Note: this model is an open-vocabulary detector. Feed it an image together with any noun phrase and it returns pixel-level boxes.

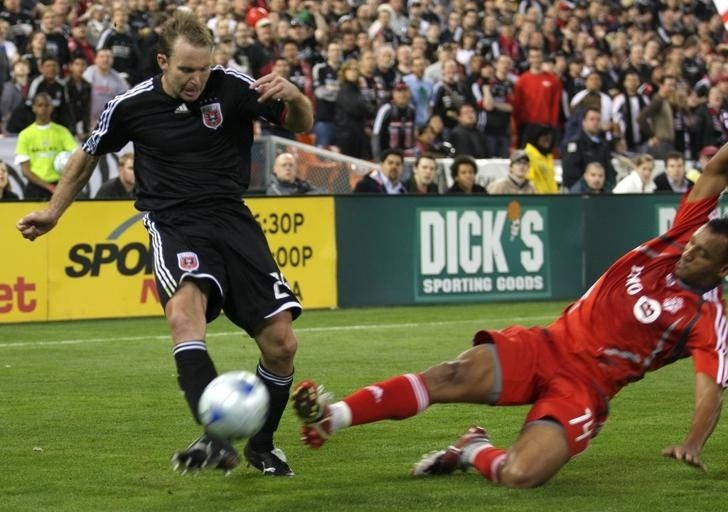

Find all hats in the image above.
[510,149,529,163]
[392,82,409,90]
[702,146,719,155]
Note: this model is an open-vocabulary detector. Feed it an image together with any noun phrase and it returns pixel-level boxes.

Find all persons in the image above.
[291,142,728,489]
[14,13,315,476]
[1,0,728,200]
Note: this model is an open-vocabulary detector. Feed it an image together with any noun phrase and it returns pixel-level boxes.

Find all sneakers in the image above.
[290,18,305,27]
[256,18,271,26]
[243,439,295,476]
[172,432,240,478]
[292,378,331,448]
[412,423,489,475]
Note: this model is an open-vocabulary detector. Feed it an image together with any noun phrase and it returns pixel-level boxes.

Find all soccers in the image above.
[198,371,270,441]
